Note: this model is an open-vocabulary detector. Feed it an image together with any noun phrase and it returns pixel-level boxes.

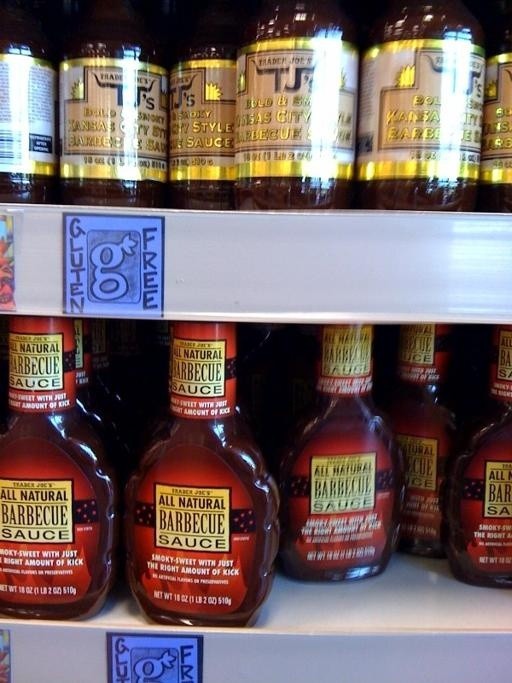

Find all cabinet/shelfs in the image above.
[0,200,511,683]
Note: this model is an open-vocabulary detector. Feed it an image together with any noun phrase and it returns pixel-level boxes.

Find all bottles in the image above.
[1,1,510,213]
[4,309,512,625]
